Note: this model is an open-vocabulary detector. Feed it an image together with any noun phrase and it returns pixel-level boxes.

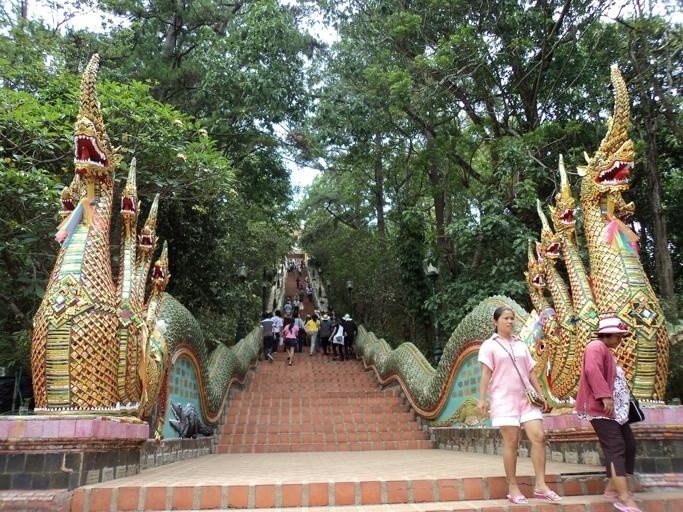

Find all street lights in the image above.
[422,246,443,366]
[345,279,355,315]
[324,279,335,312]
[234,261,247,345]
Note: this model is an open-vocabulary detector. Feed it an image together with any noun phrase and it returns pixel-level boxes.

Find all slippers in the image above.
[533,490,562,501]
[603,490,645,512]
[506,494,528,504]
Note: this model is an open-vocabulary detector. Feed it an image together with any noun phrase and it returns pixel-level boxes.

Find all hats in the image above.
[342,314,353,321]
[593,317,633,338]
[320,314,330,320]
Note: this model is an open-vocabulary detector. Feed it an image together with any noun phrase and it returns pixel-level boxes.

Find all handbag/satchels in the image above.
[627,399,645,424]
[524,391,544,408]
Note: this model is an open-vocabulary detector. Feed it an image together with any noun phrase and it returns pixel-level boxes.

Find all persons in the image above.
[258,258,357,365]
[575,317,643,511]
[475,306,561,503]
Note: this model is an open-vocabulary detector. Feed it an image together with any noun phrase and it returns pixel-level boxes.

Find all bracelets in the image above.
[539,394,545,397]
